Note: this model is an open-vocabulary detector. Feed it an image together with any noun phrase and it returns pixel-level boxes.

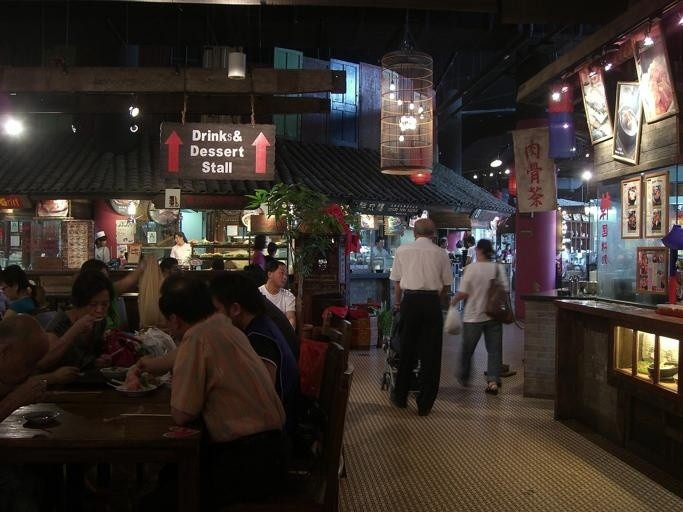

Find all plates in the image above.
[99,360,170,396]
[21,410,60,425]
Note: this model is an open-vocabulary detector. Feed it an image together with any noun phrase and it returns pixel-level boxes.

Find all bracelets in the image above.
[394,304,400,306]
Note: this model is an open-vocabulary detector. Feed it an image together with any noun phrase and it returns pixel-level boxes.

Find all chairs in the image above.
[216,319,354,512]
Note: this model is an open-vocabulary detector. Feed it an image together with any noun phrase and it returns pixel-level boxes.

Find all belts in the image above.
[404,290,438,296]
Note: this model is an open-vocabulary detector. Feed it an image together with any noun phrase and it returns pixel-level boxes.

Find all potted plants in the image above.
[243,183,362,277]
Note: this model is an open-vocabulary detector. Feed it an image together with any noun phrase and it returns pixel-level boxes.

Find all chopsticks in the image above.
[119,413,173,417]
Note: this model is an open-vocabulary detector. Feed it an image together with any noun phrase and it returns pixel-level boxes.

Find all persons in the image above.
[383,218,451,417]
[250,234,267,269]
[157,258,178,281]
[371,236,389,257]
[137,272,290,491]
[1,264,39,316]
[79,255,153,336]
[168,231,192,270]
[256,260,297,331]
[449,238,510,395]
[27,364,80,386]
[0,314,50,425]
[120,272,303,476]
[264,242,281,271]
[500,243,511,260]
[93,230,110,263]
[438,236,479,268]
[33,269,116,369]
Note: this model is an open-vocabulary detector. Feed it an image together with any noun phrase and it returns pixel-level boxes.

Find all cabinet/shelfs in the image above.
[561,213,590,277]
[191,240,287,271]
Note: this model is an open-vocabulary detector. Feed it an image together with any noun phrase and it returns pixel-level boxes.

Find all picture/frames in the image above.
[611,80,642,166]
[644,170,669,238]
[578,62,613,146]
[629,22,679,124]
[634,247,668,296]
[619,176,642,238]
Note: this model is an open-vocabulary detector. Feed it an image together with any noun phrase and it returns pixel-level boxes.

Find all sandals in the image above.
[485,384,498,393]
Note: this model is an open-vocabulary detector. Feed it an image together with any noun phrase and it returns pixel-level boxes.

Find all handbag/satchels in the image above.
[486,279,513,324]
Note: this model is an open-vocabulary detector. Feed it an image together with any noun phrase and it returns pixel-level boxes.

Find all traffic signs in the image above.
[157,124,274,180]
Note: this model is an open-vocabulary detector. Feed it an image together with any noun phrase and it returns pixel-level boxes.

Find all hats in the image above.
[95,231,105,240]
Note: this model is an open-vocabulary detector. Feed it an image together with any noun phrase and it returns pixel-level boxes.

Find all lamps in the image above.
[379,50,433,175]
[129,106,139,133]
[227,51,245,79]
[642,28,653,45]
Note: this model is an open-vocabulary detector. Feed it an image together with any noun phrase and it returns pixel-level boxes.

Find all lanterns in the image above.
[411,172,431,185]
[508,168,517,196]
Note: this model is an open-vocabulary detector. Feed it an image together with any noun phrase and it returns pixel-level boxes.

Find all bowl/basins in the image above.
[647,361,678,377]
